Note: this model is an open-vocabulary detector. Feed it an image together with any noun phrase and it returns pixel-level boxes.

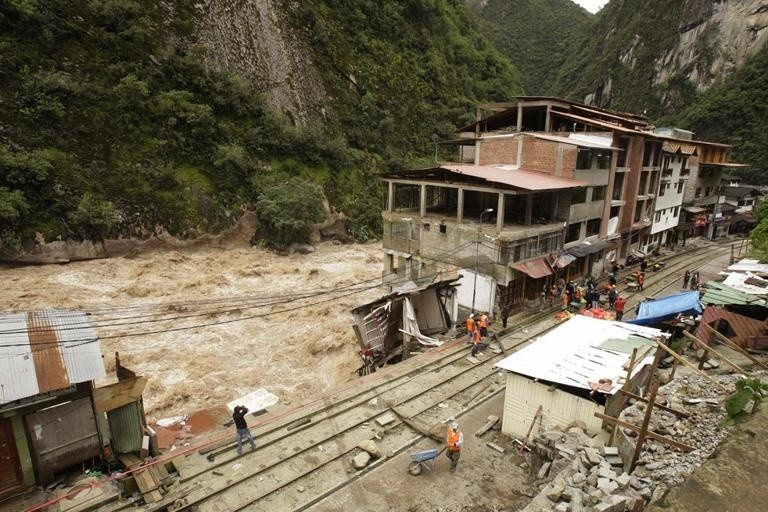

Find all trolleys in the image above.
[627,283,639,292]
[569,301,586,314]
[409,445,448,476]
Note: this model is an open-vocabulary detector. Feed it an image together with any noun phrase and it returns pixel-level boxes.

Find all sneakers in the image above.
[451,465,456,471]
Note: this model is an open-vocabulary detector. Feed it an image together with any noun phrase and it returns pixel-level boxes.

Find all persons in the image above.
[464,313,474,342]
[232,405,256,454]
[612,262,619,275]
[102,443,124,476]
[500,301,510,328]
[445,422,464,474]
[537,273,626,323]
[681,270,699,291]
[470,325,482,358]
[478,311,489,337]
[636,269,644,291]
[640,261,647,272]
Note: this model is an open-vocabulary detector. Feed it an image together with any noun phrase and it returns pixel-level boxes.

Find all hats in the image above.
[234,406,241,412]
[470,314,474,318]
[452,423,458,429]
